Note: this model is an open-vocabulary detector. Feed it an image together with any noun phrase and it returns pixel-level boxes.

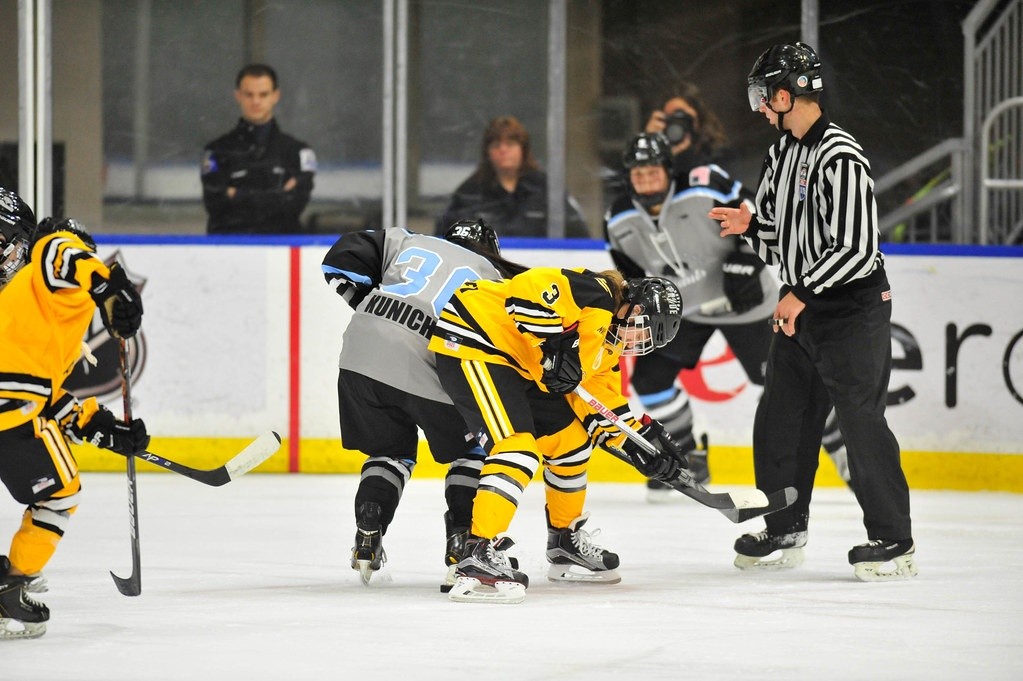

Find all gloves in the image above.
[86,260,141,339]
[622,423,689,487]
[539,321,583,395]
[721,251,767,315]
[76,403,150,458]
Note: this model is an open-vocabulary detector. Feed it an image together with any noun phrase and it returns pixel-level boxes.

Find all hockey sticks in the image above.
[84,331,145,598]
[130,428,283,488]
[539,355,799,525]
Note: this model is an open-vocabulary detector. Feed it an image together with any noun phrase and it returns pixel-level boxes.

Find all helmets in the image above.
[444,219,499,256]
[747,42,824,98]
[0,192,37,283]
[622,133,676,211]
[605,277,683,356]
[30,214,96,255]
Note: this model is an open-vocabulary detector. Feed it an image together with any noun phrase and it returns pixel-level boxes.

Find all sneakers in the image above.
[442,512,469,594]
[646,435,708,501]
[544,502,621,584]
[449,530,530,604]
[351,501,382,584]
[0,556,48,638]
[732,521,808,573]
[847,530,918,583]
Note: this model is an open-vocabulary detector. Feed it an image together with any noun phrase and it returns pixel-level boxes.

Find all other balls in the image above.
[440,584,455,593]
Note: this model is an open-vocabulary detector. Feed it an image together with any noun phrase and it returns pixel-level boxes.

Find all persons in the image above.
[434,116,594,240]
[200,61,317,235]
[604,132,858,504]
[425,262,681,603]
[322,218,532,591]
[709,43,921,584]
[0,188,152,640]
[637,80,743,178]
